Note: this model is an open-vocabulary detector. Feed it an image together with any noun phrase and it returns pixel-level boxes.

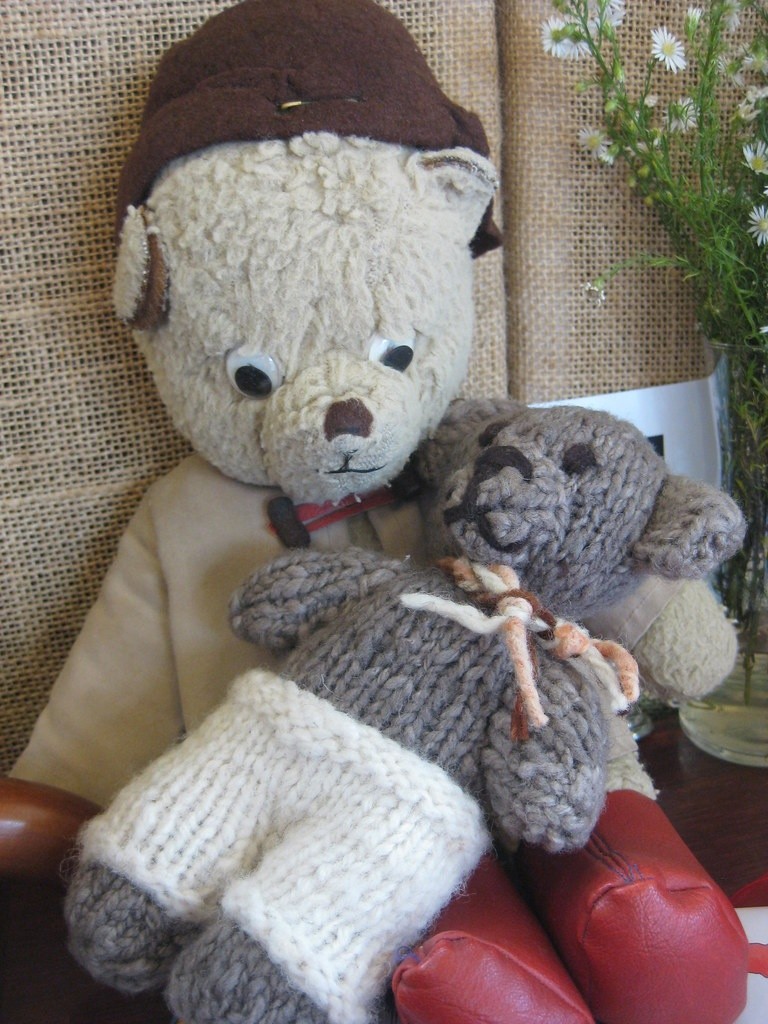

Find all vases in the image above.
[678,322,768,768]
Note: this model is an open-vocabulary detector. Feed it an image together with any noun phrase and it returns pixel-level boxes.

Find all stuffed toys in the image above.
[7,0,741,1023]
[64,396,750,1022]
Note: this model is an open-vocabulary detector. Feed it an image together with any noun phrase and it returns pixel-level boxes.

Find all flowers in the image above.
[539,0,768,350]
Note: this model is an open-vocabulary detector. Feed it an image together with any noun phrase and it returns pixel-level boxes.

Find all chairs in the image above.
[0,0,768,1024]
